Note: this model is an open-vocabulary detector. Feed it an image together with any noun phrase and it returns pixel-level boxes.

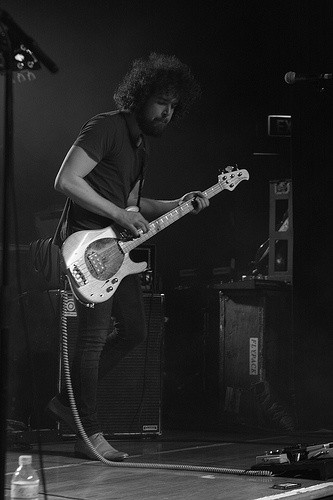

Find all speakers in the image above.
[55,290,165,442]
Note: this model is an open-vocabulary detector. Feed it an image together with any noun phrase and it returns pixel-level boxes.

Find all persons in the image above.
[53,53,211,461]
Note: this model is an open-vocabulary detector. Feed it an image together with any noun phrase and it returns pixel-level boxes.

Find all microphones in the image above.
[284,71,333,85]
[0,8,59,74]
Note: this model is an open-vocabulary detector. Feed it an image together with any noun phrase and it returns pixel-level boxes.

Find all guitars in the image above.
[59,163,249,309]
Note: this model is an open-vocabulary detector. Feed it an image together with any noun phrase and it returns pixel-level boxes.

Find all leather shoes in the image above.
[48,396,78,431]
[74,432,129,461]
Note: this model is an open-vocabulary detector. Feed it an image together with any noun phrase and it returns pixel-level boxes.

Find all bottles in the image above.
[11,455,40,500]
